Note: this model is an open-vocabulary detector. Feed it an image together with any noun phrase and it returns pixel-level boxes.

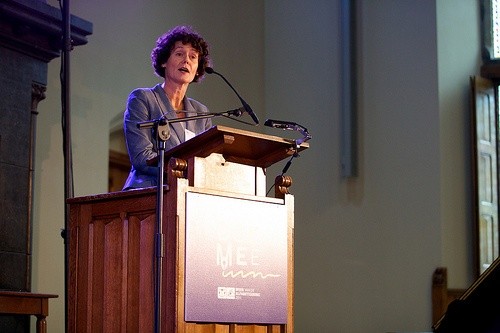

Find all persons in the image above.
[122,25,213,190]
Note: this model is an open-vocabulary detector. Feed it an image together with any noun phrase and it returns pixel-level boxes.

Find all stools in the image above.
[0,291,59,333]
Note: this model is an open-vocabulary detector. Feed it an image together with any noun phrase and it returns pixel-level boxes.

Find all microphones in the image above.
[205,66,260,125]
[264,119,297,129]
